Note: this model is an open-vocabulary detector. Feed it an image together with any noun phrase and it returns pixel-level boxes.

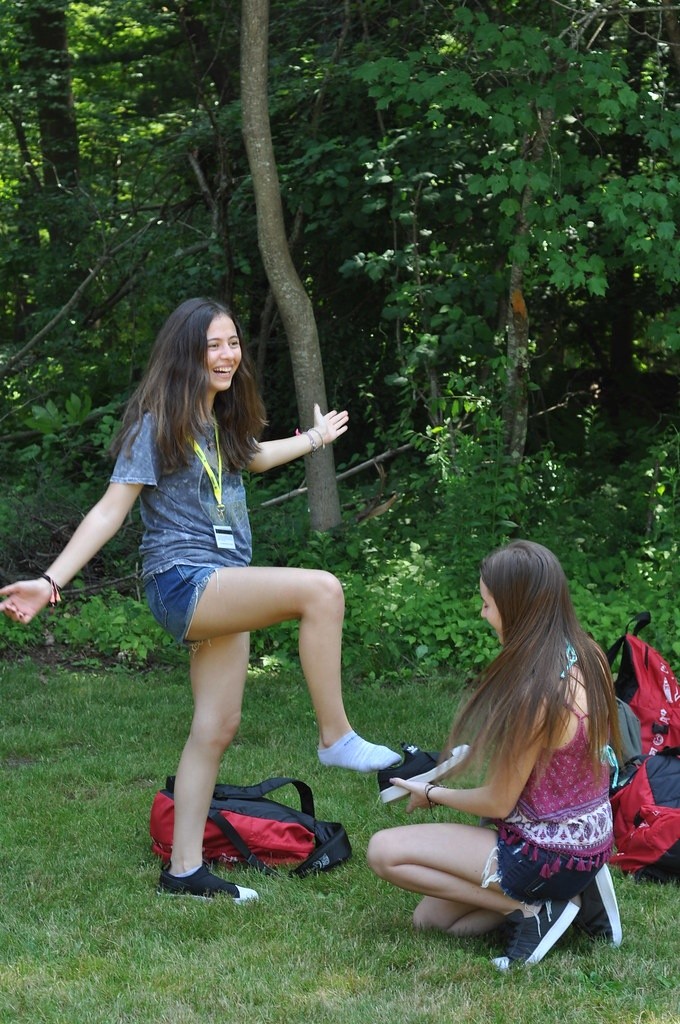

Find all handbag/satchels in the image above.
[149,772,352,876]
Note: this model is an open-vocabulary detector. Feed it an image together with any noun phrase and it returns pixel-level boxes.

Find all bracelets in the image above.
[39,571,63,609]
[301,426,327,459]
[424,782,449,821]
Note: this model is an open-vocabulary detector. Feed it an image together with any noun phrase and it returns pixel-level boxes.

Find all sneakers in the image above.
[377,741,472,802]
[571,862,623,949]
[157,859,259,905]
[491,900,580,969]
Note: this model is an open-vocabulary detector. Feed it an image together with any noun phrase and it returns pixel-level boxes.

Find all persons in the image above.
[365,536,625,977]
[0,293,405,908]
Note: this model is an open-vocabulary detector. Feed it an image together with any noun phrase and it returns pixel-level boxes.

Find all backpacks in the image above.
[601,610,680,883]
